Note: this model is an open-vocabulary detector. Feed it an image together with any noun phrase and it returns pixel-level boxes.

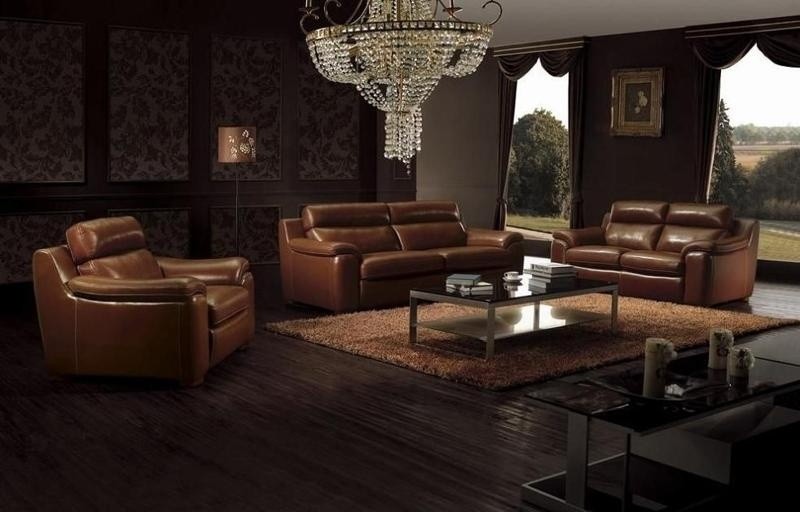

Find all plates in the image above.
[502,282,523,286]
[587,367,734,407]
[502,277,522,283]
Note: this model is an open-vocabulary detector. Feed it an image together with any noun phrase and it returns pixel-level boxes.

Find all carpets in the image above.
[263,286,799,392]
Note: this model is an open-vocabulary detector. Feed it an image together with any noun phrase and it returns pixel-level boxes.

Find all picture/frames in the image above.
[609,67,665,137]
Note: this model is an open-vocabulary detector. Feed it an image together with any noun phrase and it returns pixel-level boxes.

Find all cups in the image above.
[504,271,519,279]
[645,337,671,368]
[641,367,673,399]
[707,328,733,370]
[726,352,750,386]
[505,286,518,291]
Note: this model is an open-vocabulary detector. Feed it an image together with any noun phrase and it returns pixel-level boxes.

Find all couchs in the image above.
[550,200,759,307]
[278,202,524,313]
[33,216,256,388]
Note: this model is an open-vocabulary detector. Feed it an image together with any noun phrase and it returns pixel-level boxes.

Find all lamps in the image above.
[299,0,506,174]
[218,125,258,262]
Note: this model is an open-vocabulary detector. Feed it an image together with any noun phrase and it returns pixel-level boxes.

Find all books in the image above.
[527,260,577,290]
[446,272,494,297]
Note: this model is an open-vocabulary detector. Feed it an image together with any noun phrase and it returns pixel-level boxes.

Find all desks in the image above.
[521,328,797,511]
